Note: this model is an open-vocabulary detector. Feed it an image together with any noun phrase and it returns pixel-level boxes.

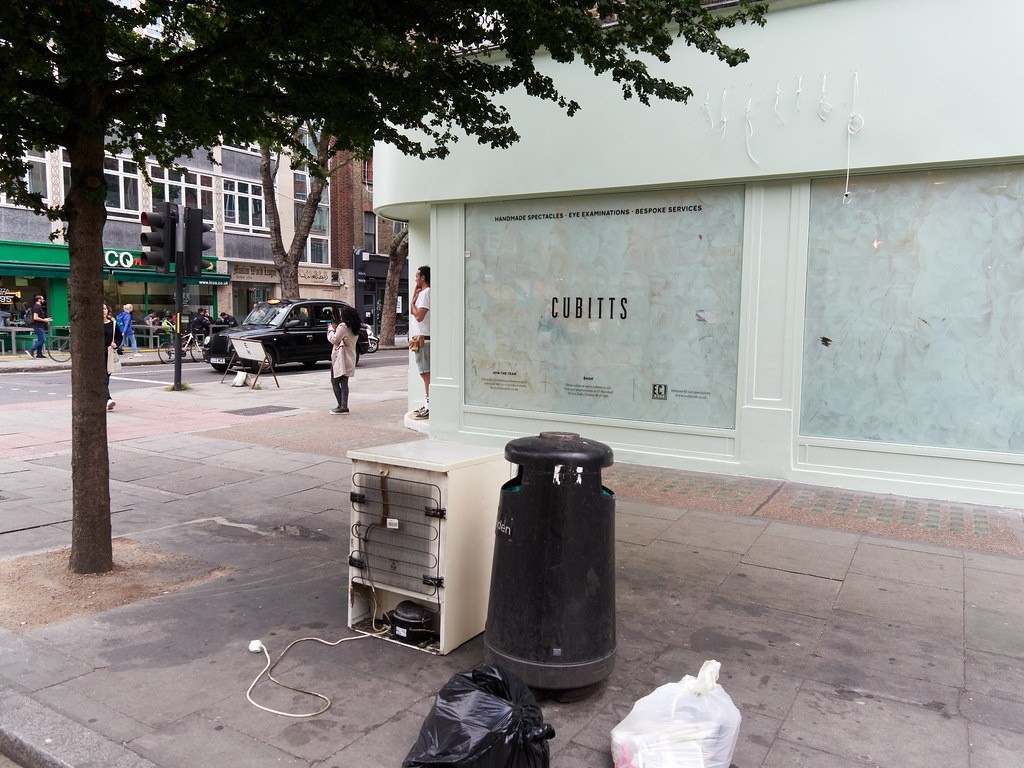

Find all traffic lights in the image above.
[185,209,212,277]
[139,202,171,274]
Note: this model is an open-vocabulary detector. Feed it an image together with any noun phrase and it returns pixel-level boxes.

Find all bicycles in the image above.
[158,327,203,364]
[47,326,72,363]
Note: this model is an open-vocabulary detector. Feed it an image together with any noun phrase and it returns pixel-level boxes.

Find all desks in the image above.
[0,326,35,356]
[49,325,71,350]
[209,324,231,335]
[132,324,170,348]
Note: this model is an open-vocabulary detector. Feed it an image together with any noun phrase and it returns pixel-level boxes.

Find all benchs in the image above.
[0,336,72,356]
[127,334,162,349]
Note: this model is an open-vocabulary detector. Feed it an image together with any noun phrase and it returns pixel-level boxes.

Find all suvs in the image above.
[202,298,369,374]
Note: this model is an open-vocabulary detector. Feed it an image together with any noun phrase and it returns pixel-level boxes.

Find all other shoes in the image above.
[36,355,47,359]
[107,399,117,411]
[134,353,143,357]
[25,349,33,358]
[117,349,123,355]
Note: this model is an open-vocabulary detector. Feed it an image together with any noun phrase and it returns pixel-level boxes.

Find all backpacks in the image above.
[22,306,37,325]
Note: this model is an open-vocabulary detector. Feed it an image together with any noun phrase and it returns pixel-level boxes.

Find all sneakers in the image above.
[330,407,349,415]
[329,405,341,412]
[413,406,426,415]
[414,408,429,420]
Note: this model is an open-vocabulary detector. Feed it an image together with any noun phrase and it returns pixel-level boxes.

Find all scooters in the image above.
[360,322,379,353]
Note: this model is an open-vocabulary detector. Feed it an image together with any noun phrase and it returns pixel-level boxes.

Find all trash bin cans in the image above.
[482,431,618,704]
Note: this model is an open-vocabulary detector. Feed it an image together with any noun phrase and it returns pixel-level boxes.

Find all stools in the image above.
[193,333,204,344]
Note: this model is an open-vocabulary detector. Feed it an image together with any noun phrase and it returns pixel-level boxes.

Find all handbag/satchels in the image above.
[408,334,425,353]
[609,659,742,768]
[107,346,122,374]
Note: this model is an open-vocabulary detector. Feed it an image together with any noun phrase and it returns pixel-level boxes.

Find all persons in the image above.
[192,308,239,338]
[410,265,430,420]
[24,295,53,359]
[0,296,29,321]
[103,301,123,410]
[116,304,144,357]
[143,311,179,333]
[327,307,366,415]
